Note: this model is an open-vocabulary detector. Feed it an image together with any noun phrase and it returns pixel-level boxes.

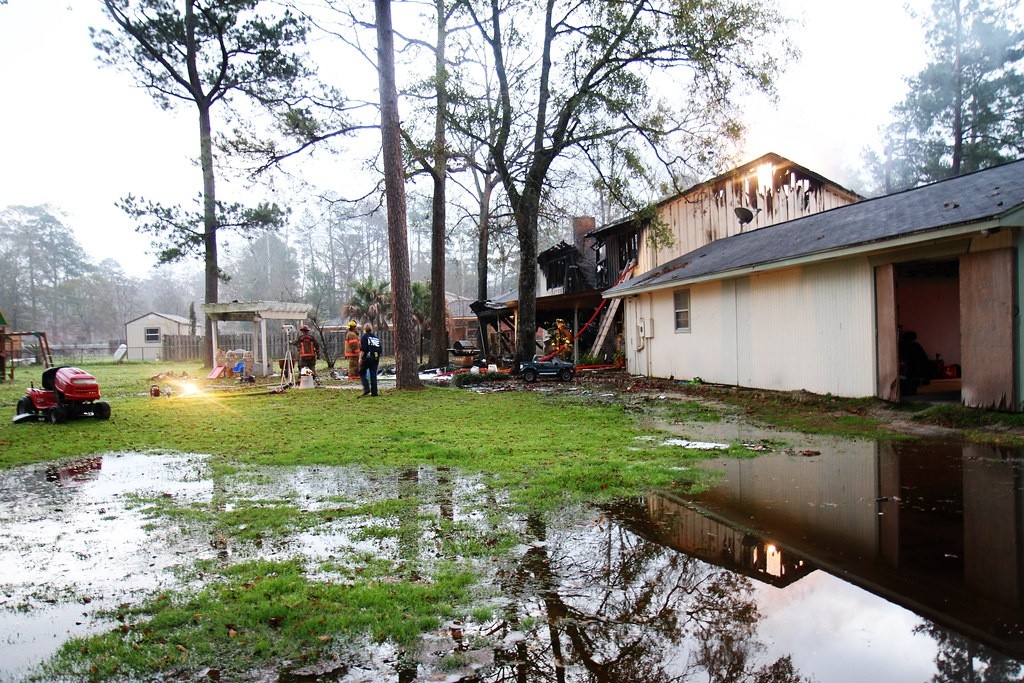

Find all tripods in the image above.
[280,335,296,388]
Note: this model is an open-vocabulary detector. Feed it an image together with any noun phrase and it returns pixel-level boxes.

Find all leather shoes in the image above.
[362,387,378,396]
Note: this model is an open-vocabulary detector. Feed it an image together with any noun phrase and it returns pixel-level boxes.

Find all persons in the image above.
[288,325,321,380]
[344,321,361,382]
[345,319,361,377]
[358,322,382,397]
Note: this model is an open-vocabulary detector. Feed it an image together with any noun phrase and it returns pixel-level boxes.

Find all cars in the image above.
[518,354,578,383]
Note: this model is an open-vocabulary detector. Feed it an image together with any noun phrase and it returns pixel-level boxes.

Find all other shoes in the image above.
[347,375,361,381]
[295,377,321,386]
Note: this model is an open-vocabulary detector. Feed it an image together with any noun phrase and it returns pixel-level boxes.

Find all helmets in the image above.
[363,323,373,331]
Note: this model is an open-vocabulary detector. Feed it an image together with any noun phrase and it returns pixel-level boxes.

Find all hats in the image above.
[556,319,564,323]
[346,320,357,329]
[299,325,310,332]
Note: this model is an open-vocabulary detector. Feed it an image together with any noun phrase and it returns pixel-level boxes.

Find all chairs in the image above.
[41,365,74,388]
